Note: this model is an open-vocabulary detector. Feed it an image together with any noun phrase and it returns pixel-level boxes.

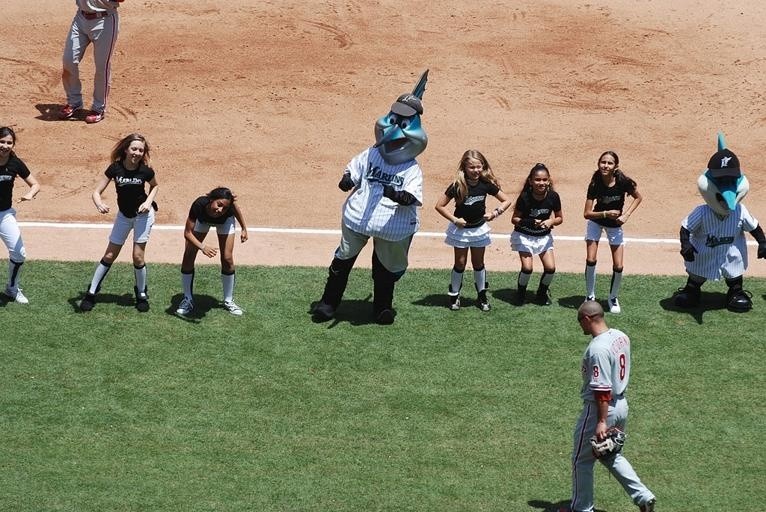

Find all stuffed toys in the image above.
[670,133,766,313]
[308,69,429,323]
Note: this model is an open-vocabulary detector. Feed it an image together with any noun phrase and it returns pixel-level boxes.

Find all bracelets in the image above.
[602,210,607,218]
[453,218,458,224]
[198,244,205,250]
[490,211,497,217]
[495,207,504,216]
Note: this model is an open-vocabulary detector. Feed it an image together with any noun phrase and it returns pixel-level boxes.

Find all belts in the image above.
[77,8,109,22]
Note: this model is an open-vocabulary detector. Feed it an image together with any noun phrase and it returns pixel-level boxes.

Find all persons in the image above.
[435,150,511,311]
[56,0,125,123]
[556,300,658,512]
[509,163,562,306]
[174,186,249,317]
[77,133,159,311]
[0,125,41,305]
[583,151,642,313]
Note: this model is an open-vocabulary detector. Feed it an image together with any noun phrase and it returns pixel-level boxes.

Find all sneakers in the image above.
[447,281,462,311]
[2,282,31,306]
[606,292,622,315]
[557,507,575,512]
[57,103,84,120]
[513,282,530,305]
[131,286,151,310]
[471,279,496,312]
[221,298,243,316]
[533,283,554,307]
[174,296,196,314]
[86,108,106,124]
[77,281,103,312]
[639,497,657,512]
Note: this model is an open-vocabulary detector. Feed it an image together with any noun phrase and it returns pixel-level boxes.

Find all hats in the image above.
[391,93,426,117]
[705,147,745,181]
